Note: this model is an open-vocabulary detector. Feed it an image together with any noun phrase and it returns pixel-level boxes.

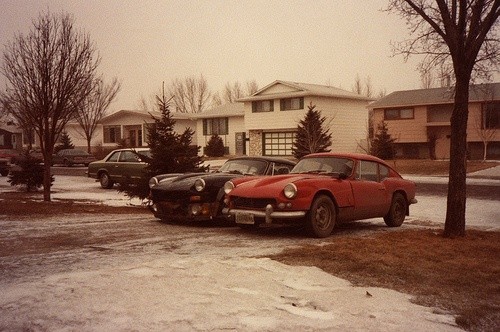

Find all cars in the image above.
[223,153,418,238]
[150,156,296,226]
[87,148,155,189]
[52,148,92,166]
